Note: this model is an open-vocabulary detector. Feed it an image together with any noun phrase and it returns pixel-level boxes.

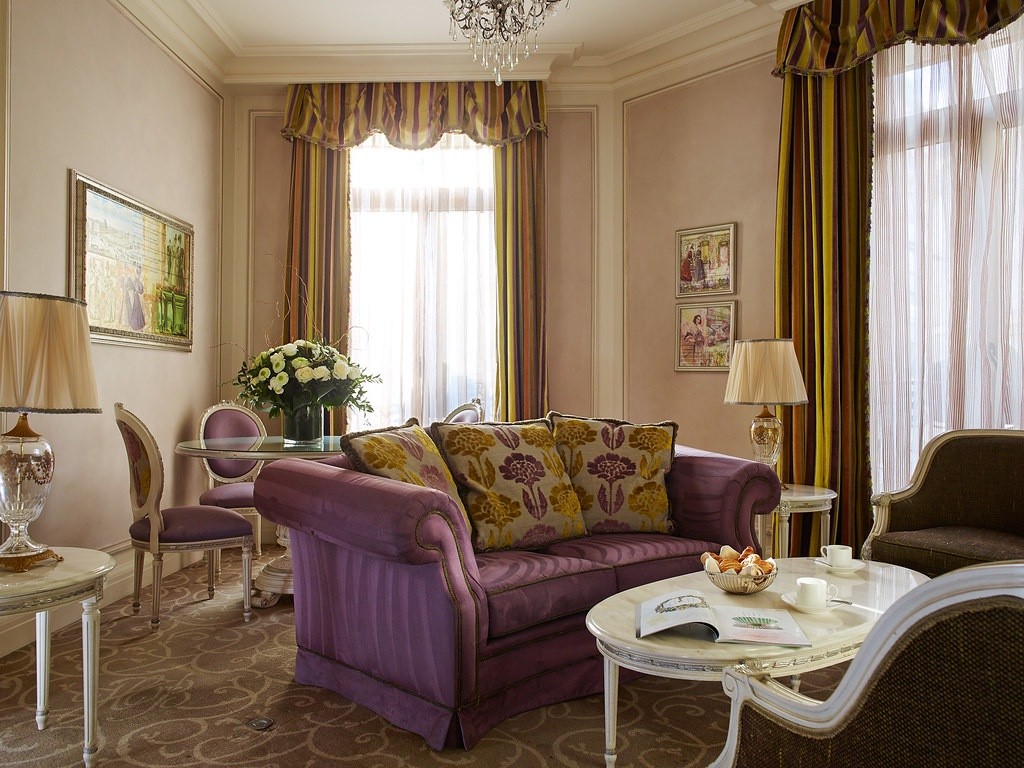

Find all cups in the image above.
[820,545,852,568]
[796,578,838,609]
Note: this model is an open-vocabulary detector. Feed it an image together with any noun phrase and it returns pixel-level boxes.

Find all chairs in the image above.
[441,398,486,424]
[112,402,255,630]
[197,400,267,561]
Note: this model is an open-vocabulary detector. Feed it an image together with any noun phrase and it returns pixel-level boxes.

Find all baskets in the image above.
[704,566,780,595]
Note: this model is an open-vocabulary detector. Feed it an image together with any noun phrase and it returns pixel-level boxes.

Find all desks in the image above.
[174,433,371,595]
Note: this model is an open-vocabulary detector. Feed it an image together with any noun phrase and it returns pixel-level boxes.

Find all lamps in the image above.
[0,288,103,575]
[723,338,809,492]
[443,0,570,86]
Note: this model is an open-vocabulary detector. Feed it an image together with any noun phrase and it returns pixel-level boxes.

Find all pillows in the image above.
[340,417,472,547]
[430,418,589,552]
[546,409,677,535]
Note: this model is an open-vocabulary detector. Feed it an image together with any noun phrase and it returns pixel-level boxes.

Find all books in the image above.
[635,591,813,648]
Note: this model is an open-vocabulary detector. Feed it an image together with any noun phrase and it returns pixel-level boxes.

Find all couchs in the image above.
[254,440,784,754]
[707,558,1024,768]
[860,428,1024,580]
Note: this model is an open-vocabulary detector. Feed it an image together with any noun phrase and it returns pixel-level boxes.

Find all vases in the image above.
[280,402,323,442]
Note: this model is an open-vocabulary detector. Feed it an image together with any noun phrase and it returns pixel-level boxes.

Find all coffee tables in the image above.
[584,556,937,768]
[754,482,837,557]
[0,545,119,757]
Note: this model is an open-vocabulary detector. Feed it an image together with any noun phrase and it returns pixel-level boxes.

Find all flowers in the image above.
[209,268,376,427]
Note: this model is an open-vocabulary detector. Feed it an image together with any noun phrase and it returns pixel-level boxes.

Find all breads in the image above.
[700,545,772,583]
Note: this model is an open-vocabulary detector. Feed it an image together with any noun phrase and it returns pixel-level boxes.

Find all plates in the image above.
[781,591,844,614]
[813,560,866,573]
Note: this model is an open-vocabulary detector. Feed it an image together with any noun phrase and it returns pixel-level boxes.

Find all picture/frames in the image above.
[672,220,739,300]
[63,166,195,356]
[674,298,739,373]
